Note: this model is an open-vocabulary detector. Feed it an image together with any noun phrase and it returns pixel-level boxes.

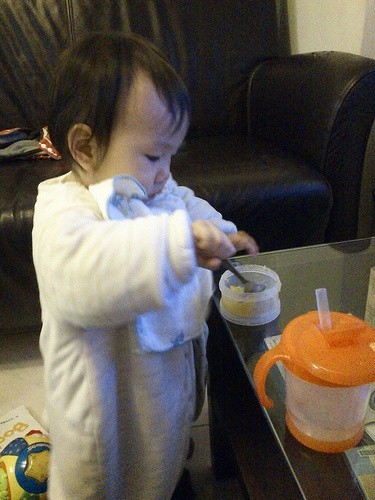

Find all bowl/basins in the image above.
[219,265,282,327]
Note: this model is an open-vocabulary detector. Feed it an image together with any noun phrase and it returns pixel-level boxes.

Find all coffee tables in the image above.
[205,238,375,500]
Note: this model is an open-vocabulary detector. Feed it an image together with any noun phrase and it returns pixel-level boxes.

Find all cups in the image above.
[253,288,375,454]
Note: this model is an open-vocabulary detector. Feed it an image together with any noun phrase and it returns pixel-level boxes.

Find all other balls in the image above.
[0,434,52,500]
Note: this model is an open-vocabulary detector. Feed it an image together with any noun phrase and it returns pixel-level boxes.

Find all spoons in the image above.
[222,258,266,292]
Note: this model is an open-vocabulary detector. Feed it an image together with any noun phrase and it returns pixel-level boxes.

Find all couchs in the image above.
[0,0,374,337]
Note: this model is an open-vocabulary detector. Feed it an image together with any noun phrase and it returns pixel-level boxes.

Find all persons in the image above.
[32,28,257,500]
